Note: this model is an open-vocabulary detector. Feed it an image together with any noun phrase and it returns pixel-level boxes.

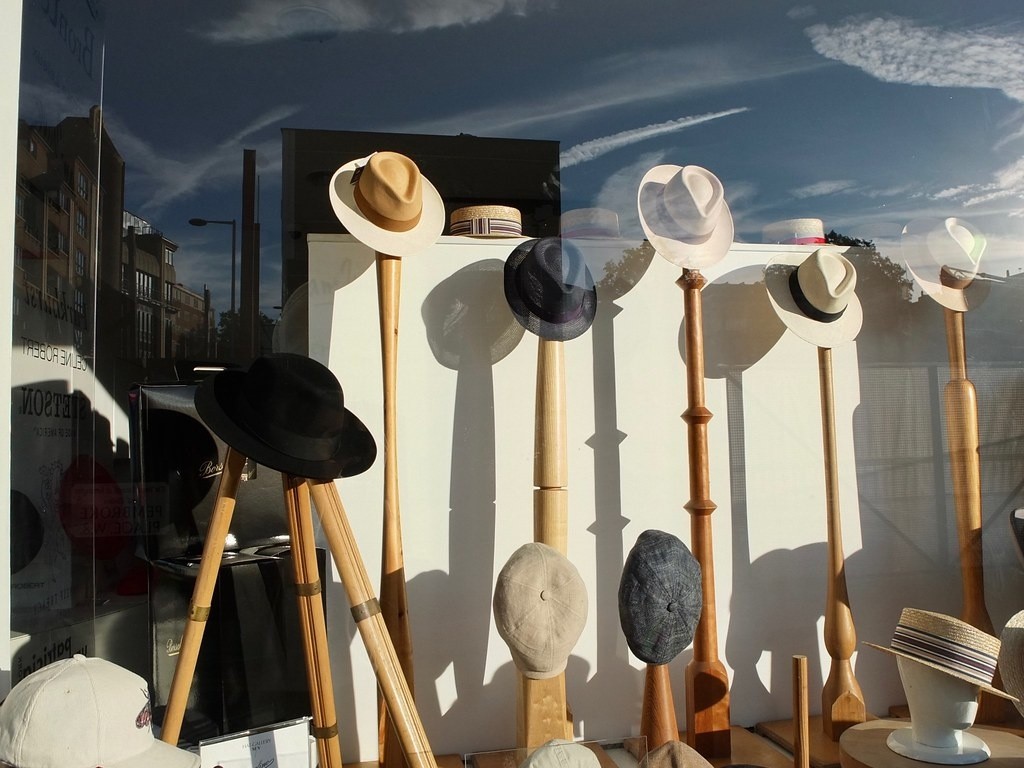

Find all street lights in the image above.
[188,217,239,362]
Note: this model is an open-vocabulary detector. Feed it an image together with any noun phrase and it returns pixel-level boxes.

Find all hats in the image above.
[272,281,309,357]
[10,489,45,573]
[503,234,599,343]
[493,542,589,680]
[900,216,992,313]
[444,205,540,238]
[519,738,601,768]
[0,653,203,768]
[557,207,631,240]
[87,409,218,528]
[637,162,735,270]
[846,223,908,246]
[56,459,125,553]
[678,263,788,380]
[860,606,1021,703]
[194,352,377,480]
[639,739,714,768]
[328,151,446,256]
[617,529,704,665]
[420,258,528,374]
[764,251,863,349]
[998,610,1024,717]
[762,218,836,246]
